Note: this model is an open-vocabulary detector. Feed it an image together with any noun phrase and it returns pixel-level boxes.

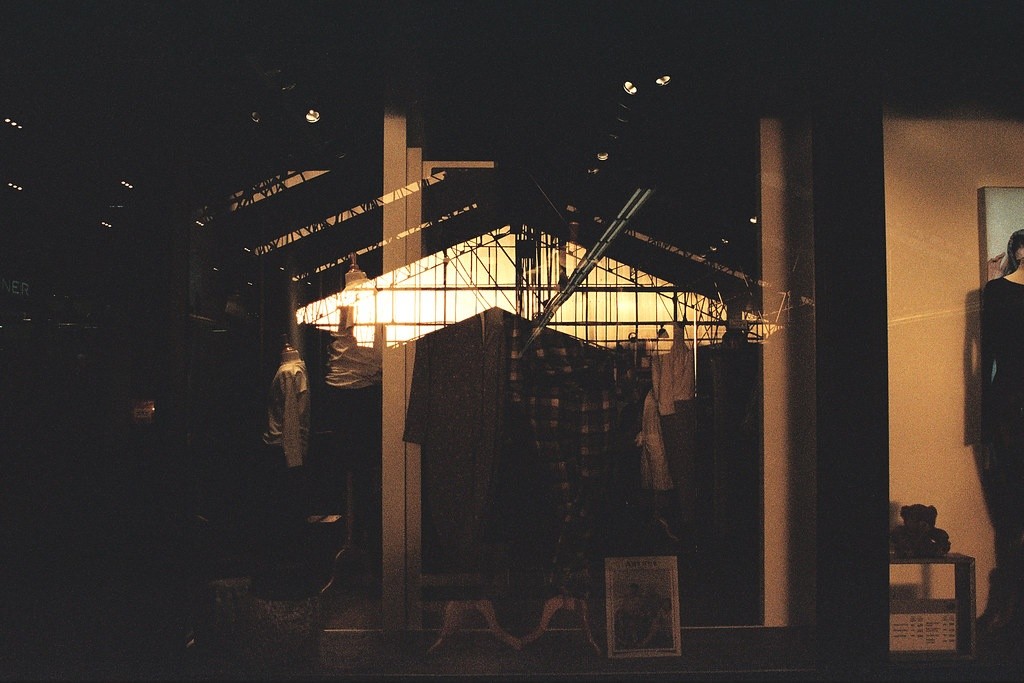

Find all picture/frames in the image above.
[600,553,688,660]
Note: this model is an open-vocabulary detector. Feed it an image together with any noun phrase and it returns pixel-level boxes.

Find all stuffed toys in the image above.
[890,504,950,557]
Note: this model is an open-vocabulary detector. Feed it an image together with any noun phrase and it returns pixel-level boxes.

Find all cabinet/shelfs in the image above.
[891,552,978,664]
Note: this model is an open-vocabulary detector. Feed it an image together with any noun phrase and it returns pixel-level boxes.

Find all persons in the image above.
[262,343,311,597]
[326,253,383,458]
